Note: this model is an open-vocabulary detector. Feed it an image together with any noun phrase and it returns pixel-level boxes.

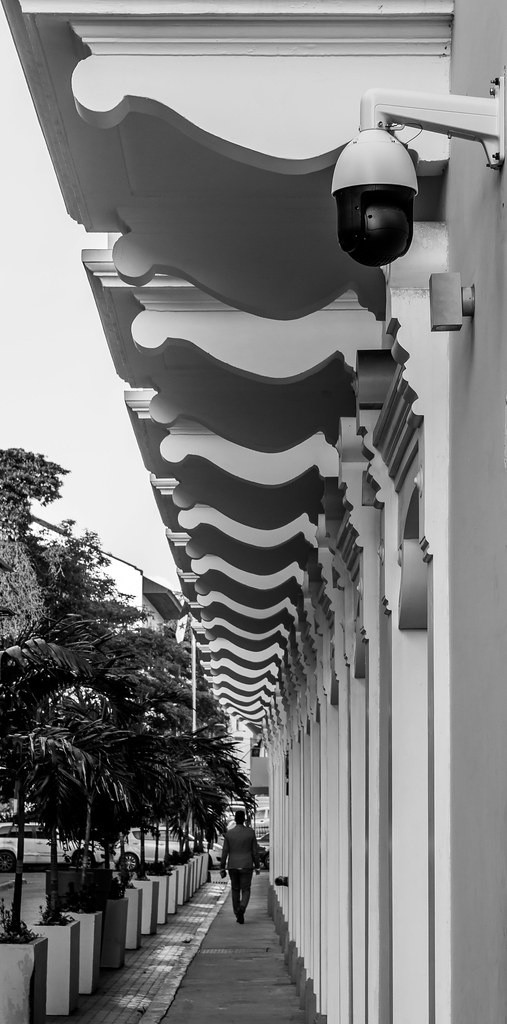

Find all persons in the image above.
[219,810,261,925]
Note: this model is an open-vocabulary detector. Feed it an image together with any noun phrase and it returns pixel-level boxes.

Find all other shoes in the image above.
[236,907,244,923]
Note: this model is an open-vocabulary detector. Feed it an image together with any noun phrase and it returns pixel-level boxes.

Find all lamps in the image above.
[429,273,474,332]
[361,470,377,507]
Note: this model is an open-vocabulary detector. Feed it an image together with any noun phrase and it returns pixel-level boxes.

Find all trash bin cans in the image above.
[45,868,109,909]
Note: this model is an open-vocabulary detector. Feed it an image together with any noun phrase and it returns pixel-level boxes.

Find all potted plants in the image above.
[0,845,209,1024]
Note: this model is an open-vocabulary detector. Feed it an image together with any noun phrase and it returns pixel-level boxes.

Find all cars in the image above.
[225,805,270,837]
[0,820,108,870]
[255,832,270,869]
[113,826,227,871]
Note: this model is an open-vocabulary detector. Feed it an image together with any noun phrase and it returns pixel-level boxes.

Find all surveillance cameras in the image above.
[330,130,418,268]
